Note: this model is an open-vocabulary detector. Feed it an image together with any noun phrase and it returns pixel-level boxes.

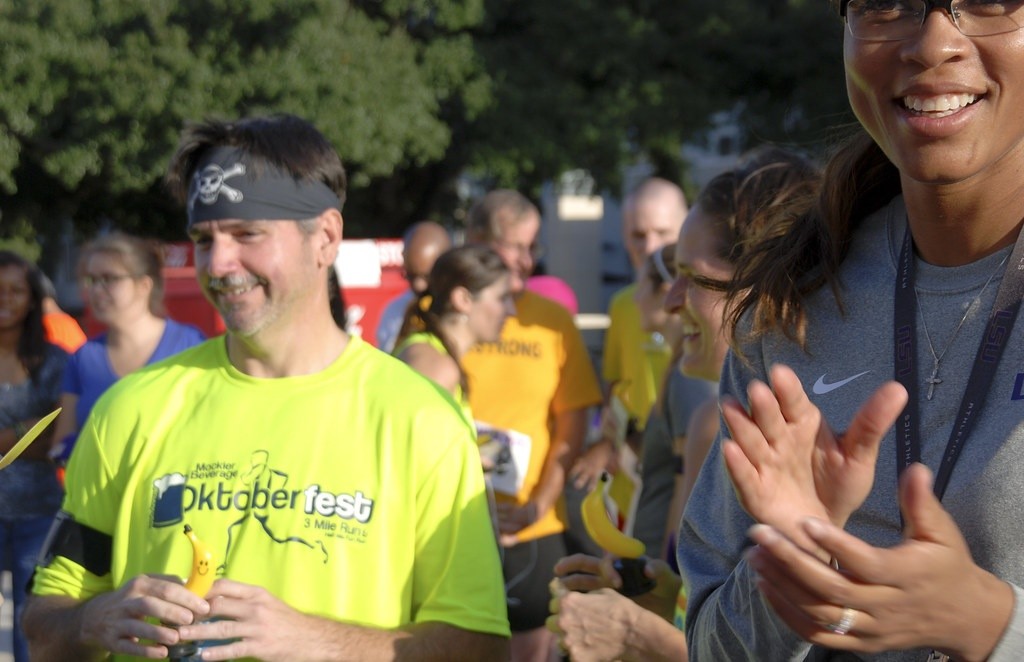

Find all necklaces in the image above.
[913,252,1009,400]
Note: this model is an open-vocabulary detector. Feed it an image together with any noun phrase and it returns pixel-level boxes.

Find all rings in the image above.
[825,608,858,635]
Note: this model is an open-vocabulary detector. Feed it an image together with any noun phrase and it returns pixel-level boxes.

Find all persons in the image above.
[675,0,1024,662]
[16,112,513,662]
[0,152,816,662]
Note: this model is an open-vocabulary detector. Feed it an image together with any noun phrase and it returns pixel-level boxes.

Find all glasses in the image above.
[841,1,1024,40]
[85,273,146,285]
